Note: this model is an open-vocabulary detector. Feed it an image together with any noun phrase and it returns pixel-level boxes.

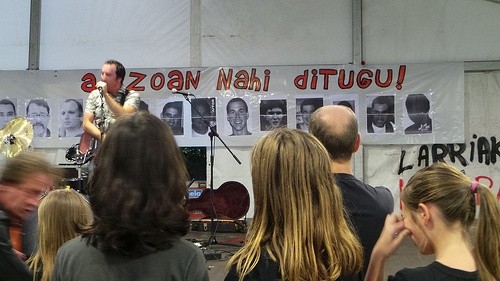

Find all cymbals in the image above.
[0,117,34,157]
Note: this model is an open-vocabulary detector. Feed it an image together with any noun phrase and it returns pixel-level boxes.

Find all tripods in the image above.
[183,95,245,250]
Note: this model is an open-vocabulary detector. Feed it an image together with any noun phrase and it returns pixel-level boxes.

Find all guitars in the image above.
[80,127,107,157]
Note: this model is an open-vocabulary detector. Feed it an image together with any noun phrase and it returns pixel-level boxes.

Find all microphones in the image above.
[98,87,102,93]
[172,88,194,96]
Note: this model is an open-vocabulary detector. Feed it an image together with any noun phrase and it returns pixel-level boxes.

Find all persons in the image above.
[260,99,286,131]
[58,99,84,137]
[0,99,17,132]
[191,99,215,137]
[222,127,363,281]
[160,102,184,135]
[81,60,140,196]
[26,99,51,137]
[363,164,500,281]
[226,98,252,136]
[51,111,210,281]
[296,99,319,132]
[367,96,394,133]
[25,189,94,281]
[310,104,395,281]
[0,151,62,281]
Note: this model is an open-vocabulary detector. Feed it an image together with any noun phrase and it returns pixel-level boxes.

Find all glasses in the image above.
[29,113,49,119]
[13,184,48,201]
